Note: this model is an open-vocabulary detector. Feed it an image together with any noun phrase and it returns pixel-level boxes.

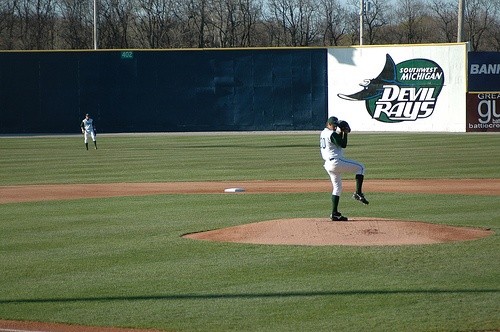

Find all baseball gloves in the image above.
[332,119,352,133]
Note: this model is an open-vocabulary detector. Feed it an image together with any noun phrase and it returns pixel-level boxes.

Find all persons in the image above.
[80,113,99,151]
[320,116,368,221]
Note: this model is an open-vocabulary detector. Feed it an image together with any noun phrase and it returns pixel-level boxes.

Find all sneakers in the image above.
[330,211,348,221]
[352,192,369,205]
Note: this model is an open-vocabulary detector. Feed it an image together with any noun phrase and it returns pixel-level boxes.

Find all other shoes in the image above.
[94,146,97,150]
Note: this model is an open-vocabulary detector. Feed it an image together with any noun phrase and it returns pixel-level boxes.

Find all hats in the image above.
[86,114,90,116]
[328,116,339,127]
